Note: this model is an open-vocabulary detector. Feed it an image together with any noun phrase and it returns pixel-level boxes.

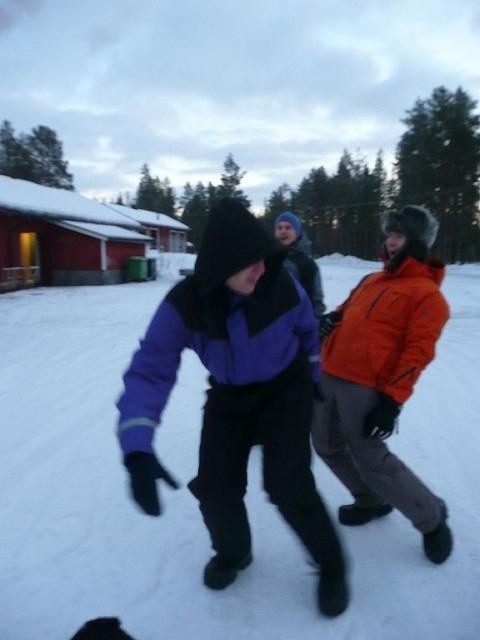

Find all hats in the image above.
[382,204,439,272]
[276,212,303,239]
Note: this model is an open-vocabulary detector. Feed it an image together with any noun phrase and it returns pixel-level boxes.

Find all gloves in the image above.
[123,449,179,517]
[319,311,341,341]
[362,391,401,442]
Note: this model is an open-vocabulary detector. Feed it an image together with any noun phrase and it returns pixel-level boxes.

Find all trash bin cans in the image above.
[127,256,147,280]
[148,258,156,280]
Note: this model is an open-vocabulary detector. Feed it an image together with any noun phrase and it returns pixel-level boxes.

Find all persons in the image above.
[311,204,455,565]
[265,209,327,320]
[115,201,351,617]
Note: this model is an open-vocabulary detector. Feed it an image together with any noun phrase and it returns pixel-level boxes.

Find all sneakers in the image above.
[204,551,252,591]
[339,502,390,527]
[423,499,453,564]
[317,559,348,618]
